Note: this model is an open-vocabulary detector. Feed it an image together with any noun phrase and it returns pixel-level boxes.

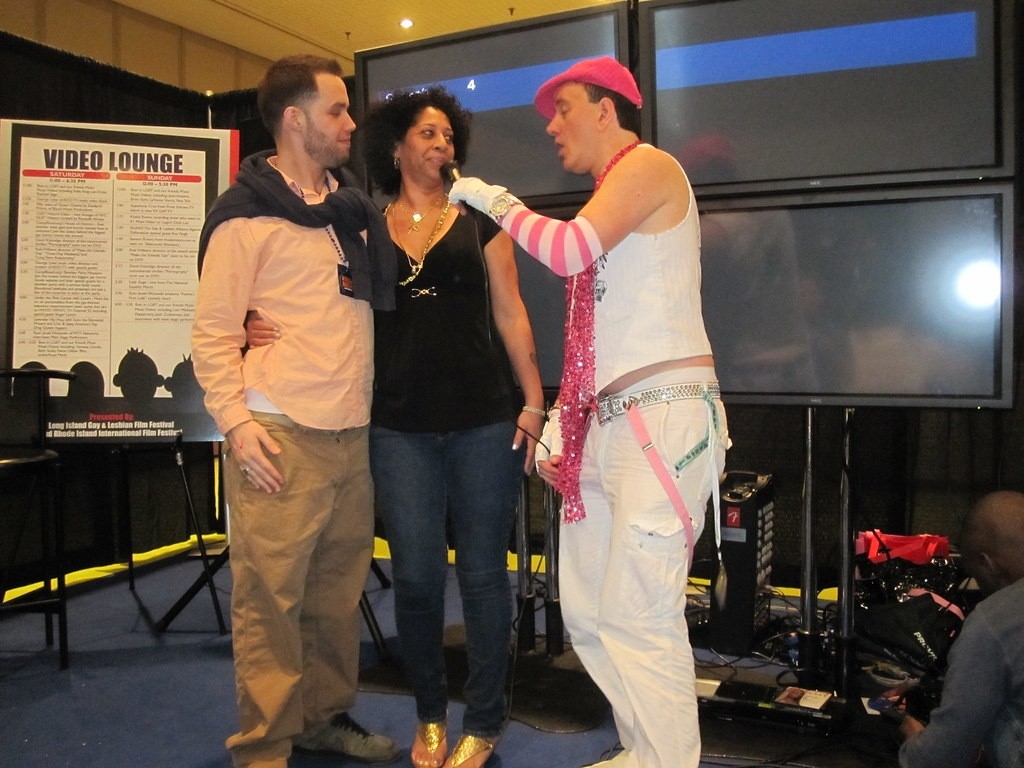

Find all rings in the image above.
[243,468,248,475]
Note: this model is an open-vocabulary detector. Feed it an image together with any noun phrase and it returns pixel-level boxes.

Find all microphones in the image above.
[440,158,479,224]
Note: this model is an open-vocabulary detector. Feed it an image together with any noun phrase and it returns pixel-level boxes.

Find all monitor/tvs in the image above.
[351,0,1024,408]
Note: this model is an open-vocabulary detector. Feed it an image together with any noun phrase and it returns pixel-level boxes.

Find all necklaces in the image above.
[300,178,348,262]
[401,204,437,233]
[392,200,449,288]
[554,140,645,521]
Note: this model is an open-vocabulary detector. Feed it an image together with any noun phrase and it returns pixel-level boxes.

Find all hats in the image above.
[535,57,643,119]
[678,135,734,174]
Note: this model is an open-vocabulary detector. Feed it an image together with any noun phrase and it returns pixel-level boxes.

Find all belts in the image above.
[596,381,720,423]
[249,411,297,432]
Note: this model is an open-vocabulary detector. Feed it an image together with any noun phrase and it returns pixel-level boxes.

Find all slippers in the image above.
[411,722,448,768]
[443,734,501,768]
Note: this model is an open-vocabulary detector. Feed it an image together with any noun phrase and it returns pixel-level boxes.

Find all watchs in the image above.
[488,194,522,223]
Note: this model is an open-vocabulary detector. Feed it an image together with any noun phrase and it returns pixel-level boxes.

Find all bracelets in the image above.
[522,406,545,416]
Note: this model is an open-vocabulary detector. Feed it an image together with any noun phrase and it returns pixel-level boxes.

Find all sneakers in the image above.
[292,711,402,765]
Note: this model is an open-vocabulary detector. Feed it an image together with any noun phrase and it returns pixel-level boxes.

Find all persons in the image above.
[190,56,399,767]
[899,490,1023,768]
[241,88,543,767]
[447,57,732,767]
